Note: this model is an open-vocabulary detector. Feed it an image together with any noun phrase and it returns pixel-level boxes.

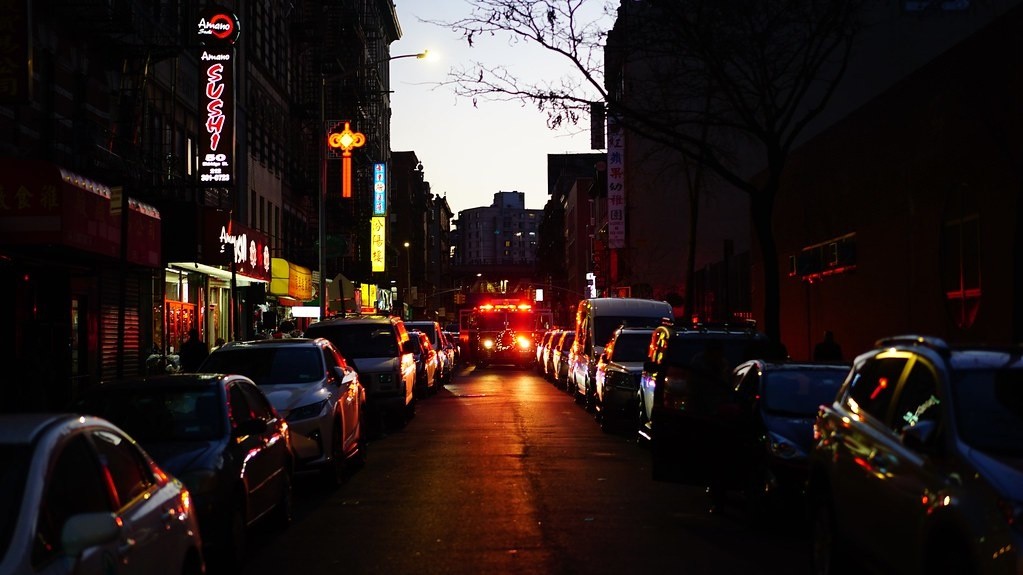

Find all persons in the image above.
[814,330,843,363]
[210,320,306,355]
[179,329,209,373]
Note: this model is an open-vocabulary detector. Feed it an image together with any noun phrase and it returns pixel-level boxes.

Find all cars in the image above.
[59,367,302,575]
[0,409,207,575]
[532,328,576,388]
[692,356,853,535]
[402,315,465,399]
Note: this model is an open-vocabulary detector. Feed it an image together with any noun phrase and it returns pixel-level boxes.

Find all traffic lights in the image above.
[453,293,460,305]
[531,289,536,299]
[461,294,466,304]
[524,289,530,299]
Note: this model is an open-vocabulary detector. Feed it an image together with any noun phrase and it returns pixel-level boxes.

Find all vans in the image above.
[567,296,679,413]
[301,315,417,434]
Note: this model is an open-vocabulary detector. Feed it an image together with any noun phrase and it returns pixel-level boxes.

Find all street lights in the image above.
[403,241,414,306]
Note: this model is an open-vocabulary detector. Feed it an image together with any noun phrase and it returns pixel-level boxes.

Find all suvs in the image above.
[799,335,1023,575]
[630,317,799,484]
[176,335,367,482]
[596,321,658,437]
